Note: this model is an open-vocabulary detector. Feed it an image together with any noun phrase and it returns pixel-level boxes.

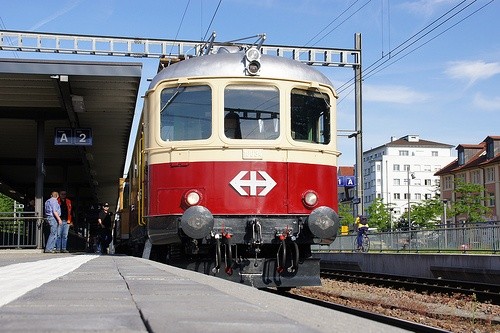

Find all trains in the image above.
[112,33,340,294]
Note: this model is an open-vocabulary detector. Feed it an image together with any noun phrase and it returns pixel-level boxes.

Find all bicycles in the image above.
[352,222,370,253]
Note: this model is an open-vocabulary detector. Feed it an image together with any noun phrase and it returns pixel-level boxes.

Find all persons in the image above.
[356,213,369,249]
[54,190,73,253]
[43,191,63,253]
[85,201,125,256]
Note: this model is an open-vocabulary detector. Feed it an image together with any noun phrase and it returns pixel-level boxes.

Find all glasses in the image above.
[104,205,110,207]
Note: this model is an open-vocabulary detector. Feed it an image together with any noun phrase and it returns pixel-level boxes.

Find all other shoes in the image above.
[45,250,69,253]
